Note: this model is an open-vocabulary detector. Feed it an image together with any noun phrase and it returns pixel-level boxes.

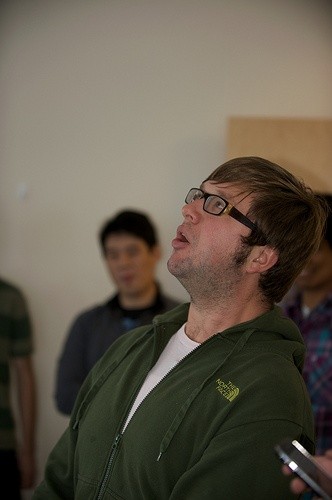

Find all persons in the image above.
[286,193,332,458]
[52,207,185,420]
[0,279,41,500]
[33,156,325,500]
[274,438,332,500]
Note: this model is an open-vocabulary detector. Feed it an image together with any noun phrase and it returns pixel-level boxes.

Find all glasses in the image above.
[184,188,265,237]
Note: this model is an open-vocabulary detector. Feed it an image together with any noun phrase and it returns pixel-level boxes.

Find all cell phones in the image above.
[273,435,332,500]
